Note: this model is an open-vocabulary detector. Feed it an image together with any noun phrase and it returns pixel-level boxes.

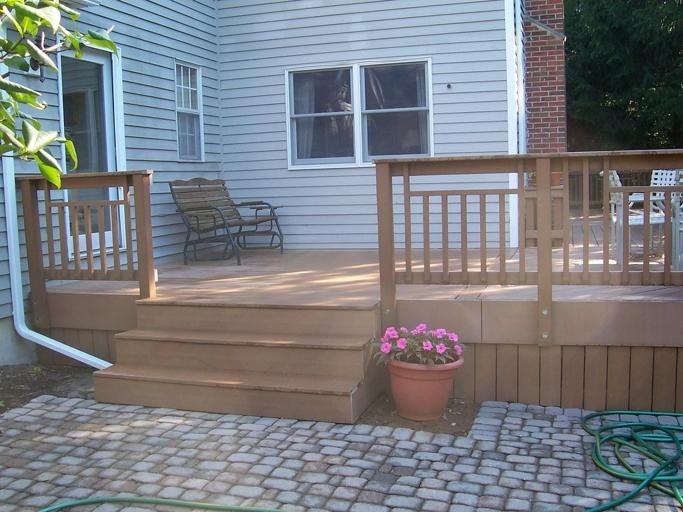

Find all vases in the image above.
[387,361,461,422]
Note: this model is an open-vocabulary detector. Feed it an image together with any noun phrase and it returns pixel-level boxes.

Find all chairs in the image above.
[600,169,682,270]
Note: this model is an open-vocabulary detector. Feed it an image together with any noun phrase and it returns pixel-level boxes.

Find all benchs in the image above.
[169,178,285,267]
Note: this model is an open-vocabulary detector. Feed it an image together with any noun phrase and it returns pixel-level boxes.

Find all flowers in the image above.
[372,322,462,369]
